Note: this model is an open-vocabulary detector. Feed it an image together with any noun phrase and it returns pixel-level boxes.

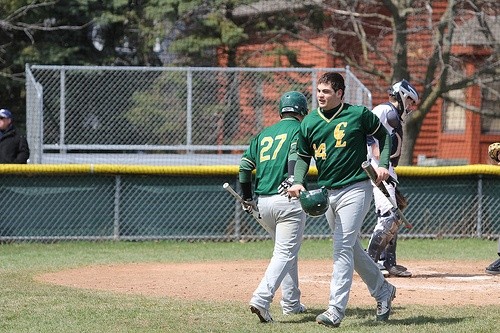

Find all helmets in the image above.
[299,186,330,216]
[278,91,308,118]
[384,78,420,122]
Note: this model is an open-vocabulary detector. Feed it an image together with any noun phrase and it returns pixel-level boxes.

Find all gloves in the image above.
[241,199,258,215]
[385,175,399,187]
[278,174,294,202]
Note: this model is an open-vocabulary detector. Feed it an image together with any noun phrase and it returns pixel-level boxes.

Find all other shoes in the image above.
[250,306,273,322]
[485,258,500,274]
[298,303,305,313]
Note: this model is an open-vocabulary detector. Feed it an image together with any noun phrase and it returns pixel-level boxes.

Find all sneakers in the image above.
[388,265,412,277]
[380,268,389,277]
[376,286,396,321]
[316,309,341,328]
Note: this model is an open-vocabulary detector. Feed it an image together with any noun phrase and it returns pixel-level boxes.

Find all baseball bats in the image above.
[224,183,273,235]
[362,160,412,229]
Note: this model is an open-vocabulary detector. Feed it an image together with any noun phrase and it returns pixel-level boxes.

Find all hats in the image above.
[0,109,11,117]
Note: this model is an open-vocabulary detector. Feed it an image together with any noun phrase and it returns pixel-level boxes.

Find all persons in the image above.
[238,91,309,323]
[281,72,396,328]
[365,80,419,278]
[0,109,32,164]
[484,142,499,275]
